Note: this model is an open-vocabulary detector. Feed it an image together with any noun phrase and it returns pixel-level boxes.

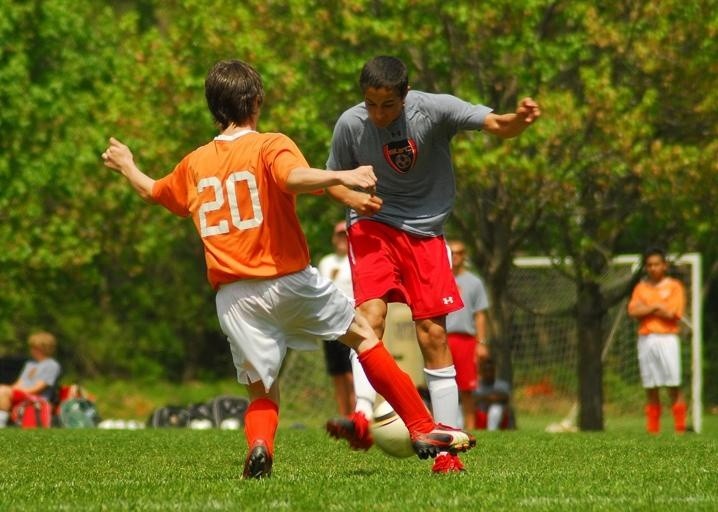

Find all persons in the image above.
[322,53,543,476]
[102,57,477,481]
[2,330,63,428]
[443,240,493,433]
[316,212,356,417]
[626,247,690,434]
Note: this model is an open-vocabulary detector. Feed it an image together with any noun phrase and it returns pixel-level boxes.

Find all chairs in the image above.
[9,381,100,431]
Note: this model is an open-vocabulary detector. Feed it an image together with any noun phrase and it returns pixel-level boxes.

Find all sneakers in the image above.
[431,452,466,474]
[243,439,273,478]
[409,424,477,459]
[327,412,373,450]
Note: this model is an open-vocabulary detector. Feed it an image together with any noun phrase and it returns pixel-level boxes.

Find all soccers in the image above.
[370,398,432,460]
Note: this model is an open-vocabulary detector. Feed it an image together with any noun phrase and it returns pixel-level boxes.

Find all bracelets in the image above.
[479,338,489,345]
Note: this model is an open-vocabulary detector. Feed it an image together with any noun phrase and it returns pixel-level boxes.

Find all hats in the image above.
[12,390,37,403]
